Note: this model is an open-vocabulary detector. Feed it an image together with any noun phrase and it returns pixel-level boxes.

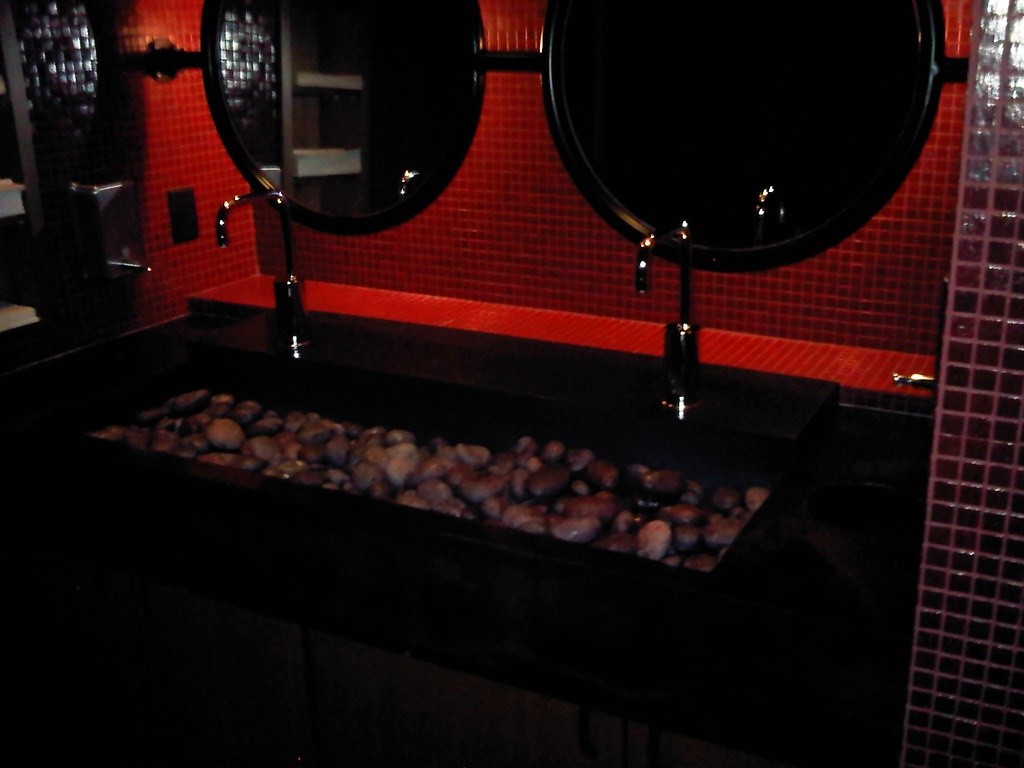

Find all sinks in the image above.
[5,327,796,577]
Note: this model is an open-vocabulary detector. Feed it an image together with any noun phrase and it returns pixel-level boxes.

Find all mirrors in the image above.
[541,1,943,272]
[199,0,486,235]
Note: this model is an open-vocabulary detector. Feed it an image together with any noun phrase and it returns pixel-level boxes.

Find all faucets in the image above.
[631,224,698,402]
[398,168,421,199]
[212,186,316,347]
[755,182,787,244]
